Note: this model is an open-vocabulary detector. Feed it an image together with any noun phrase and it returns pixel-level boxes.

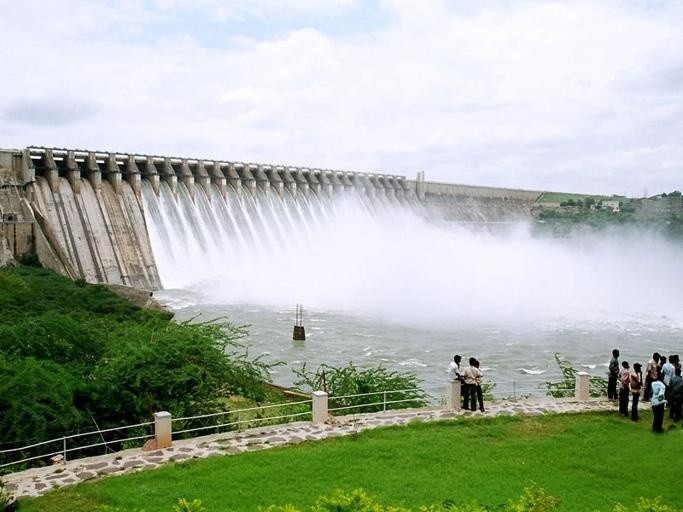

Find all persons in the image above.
[145,291,155,309]
[448,355,485,413]
[609,349,683,433]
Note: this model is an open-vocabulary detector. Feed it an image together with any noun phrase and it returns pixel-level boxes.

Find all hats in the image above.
[632,363,642,368]
